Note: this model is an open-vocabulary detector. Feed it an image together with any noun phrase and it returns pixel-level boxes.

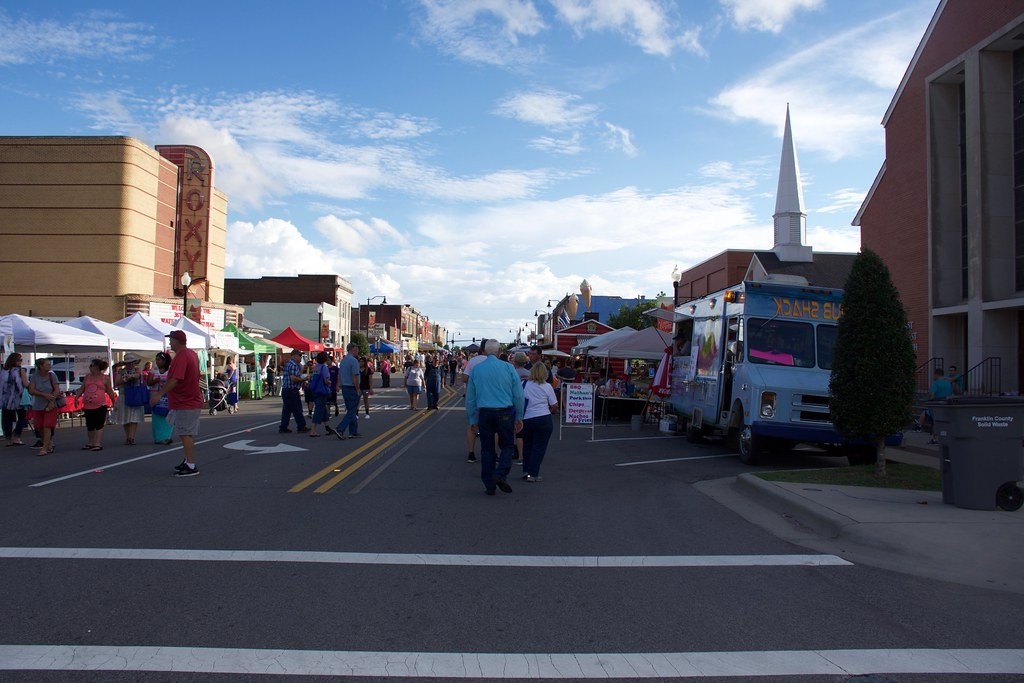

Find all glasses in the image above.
[159,352,166,359]
[296,354,302,357]
[89,364,96,367]
[18,359,23,362]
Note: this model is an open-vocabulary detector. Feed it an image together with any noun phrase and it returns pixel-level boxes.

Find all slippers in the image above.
[310,434,321,437]
[326,430,333,435]
[82,444,103,451]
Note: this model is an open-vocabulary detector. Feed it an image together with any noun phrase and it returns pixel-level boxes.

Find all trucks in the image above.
[641,278,904,468]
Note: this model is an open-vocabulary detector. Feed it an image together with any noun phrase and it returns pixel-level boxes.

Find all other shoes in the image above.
[30,440,44,448]
[512,459,522,465]
[125,438,136,445]
[6,439,26,446]
[482,474,512,495]
[524,472,543,482]
[234,406,239,413]
[165,439,173,445]
[297,427,311,433]
[38,447,53,455]
[279,428,292,433]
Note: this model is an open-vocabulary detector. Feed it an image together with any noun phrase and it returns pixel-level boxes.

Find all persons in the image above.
[672,333,691,356]
[0,330,560,497]
[914,355,963,447]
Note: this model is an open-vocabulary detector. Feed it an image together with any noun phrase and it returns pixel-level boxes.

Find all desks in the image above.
[239,380,263,399]
[276,374,309,396]
[598,396,661,426]
[26,392,117,431]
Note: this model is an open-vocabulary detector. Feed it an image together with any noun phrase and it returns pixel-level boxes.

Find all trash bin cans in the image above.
[924,397,1024,511]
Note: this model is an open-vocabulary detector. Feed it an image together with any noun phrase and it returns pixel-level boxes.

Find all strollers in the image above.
[208,372,235,416]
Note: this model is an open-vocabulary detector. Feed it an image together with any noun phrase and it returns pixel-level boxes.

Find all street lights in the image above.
[671,264,681,308]
[366,295,387,340]
[180,272,192,317]
[525,321,536,345]
[534,310,551,342]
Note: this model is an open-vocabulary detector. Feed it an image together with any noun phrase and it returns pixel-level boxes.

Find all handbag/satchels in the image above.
[105,391,118,407]
[310,365,333,395]
[19,385,32,406]
[125,374,150,407]
[54,395,67,408]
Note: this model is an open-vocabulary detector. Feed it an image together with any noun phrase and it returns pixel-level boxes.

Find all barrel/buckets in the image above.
[631,415,643,431]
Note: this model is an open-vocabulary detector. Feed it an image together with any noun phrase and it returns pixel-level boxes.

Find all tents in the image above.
[0,306,402,428]
[459,325,672,427]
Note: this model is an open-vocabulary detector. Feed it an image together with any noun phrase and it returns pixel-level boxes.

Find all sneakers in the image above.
[355,415,359,420]
[334,428,346,440]
[172,458,200,477]
[467,452,478,462]
[364,414,370,419]
[348,433,364,438]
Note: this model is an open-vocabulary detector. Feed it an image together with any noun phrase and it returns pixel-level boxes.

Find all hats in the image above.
[347,343,361,351]
[164,330,186,342]
[291,350,305,356]
[120,354,140,364]
[510,352,526,361]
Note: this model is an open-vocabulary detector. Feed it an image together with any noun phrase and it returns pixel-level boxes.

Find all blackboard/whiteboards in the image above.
[565,384,593,424]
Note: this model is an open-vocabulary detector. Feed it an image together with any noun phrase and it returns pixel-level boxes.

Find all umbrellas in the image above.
[641,344,674,415]
